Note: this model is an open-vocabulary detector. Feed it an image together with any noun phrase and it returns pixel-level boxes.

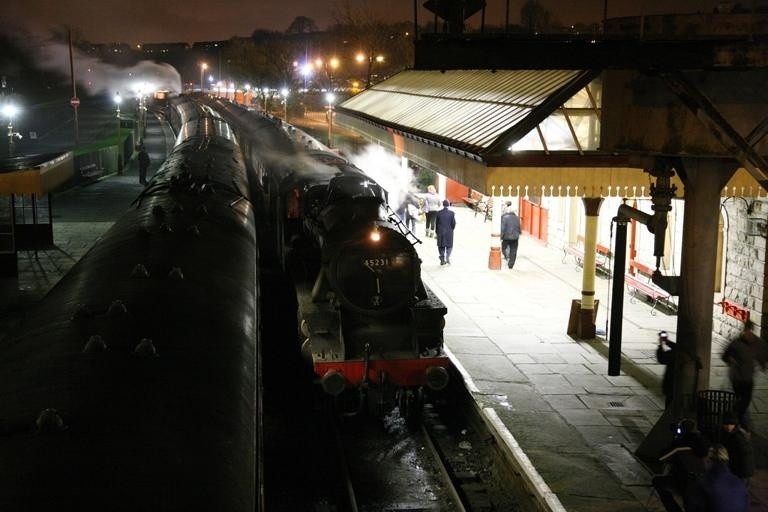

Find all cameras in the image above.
[659,331,667,341]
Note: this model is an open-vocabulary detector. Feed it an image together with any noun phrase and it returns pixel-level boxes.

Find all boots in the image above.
[439,255,446,265]
[425,227,435,238]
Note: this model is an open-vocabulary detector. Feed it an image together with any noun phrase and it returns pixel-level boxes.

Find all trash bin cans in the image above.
[695,390,745,447]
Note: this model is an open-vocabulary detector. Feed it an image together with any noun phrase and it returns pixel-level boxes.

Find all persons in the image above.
[502,199,514,257]
[720,319,768,422]
[395,204,405,222]
[650,416,703,512]
[500,206,521,271]
[437,198,456,267]
[406,192,419,237]
[424,185,440,239]
[685,442,751,512]
[138,146,151,186]
[710,413,757,480]
[655,331,702,411]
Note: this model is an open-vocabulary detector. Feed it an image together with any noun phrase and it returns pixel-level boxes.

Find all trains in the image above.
[188,85,453,423]
[0,93,269,508]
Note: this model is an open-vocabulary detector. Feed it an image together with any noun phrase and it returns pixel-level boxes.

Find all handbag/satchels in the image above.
[422,196,430,214]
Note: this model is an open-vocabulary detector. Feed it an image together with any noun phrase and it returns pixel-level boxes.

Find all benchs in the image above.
[561,233,677,317]
[77,163,106,184]
[461,186,494,225]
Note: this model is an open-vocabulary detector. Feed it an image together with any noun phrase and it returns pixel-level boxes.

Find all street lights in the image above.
[314,57,339,91]
[2,101,18,159]
[115,93,122,142]
[325,92,334,148]
[131,82,154,147]
[355,52,386,90]
[278,86,290,123]
[199,61,268,110]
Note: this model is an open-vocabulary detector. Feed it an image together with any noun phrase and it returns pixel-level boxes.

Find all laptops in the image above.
[674,440,708,479]
